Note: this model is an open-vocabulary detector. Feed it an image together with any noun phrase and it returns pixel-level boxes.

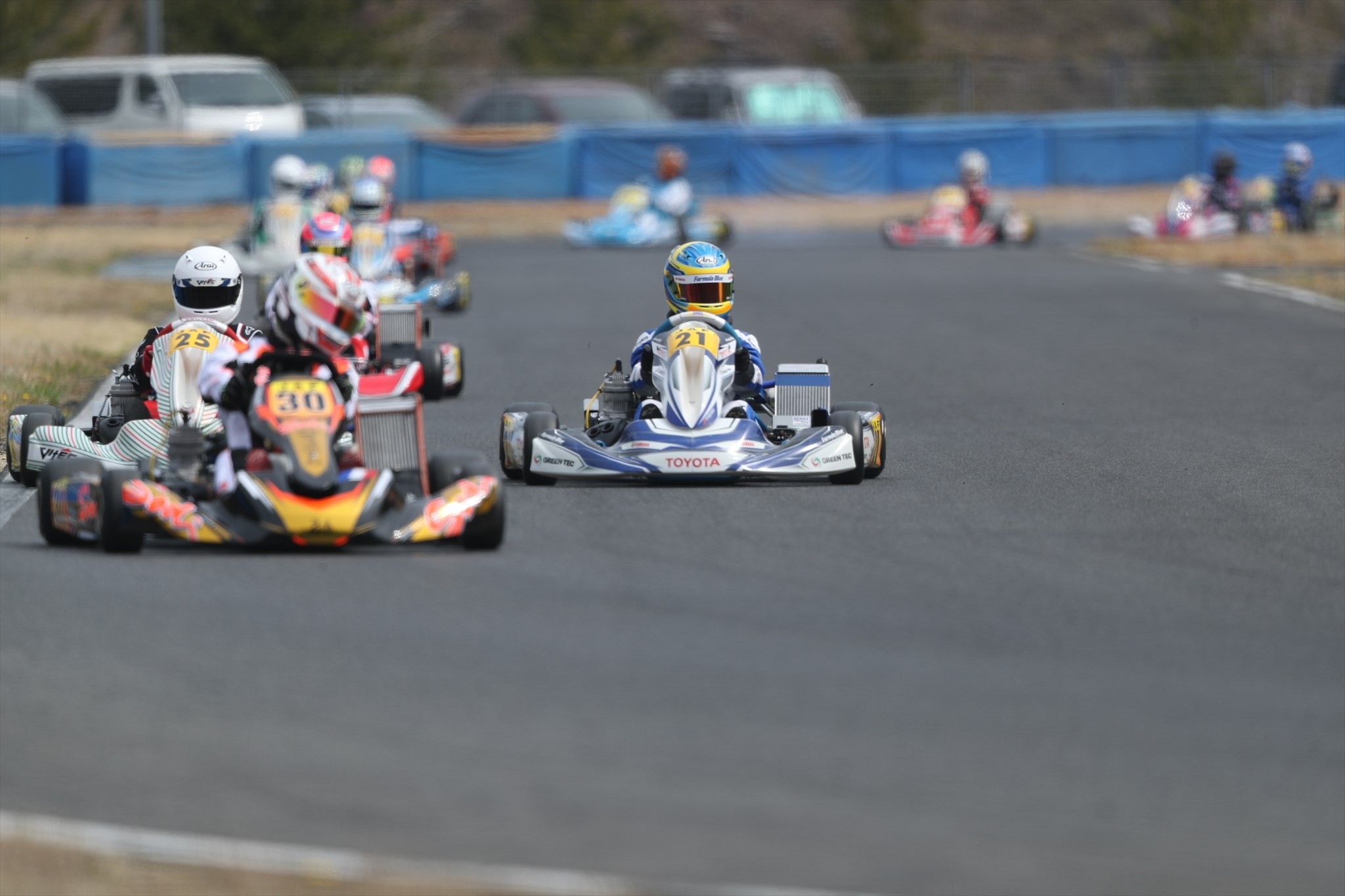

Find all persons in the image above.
[632,242,762,419]
[958,145,989,226]
[125,245,265,434]
[1203,139,1312,212]
[612,146,695,218]
[262,215,376,337]
[228,153,397,278]
[199,251,363,470]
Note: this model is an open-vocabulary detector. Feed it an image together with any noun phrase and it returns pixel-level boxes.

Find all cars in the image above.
[454,79,671,129]
[1,75,65,140]
[304,93,451,133]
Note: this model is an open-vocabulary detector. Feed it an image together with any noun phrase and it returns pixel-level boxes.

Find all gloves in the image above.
[217,362,255,413]
[733,346,755,386]
[640,344,654,386]
[141,344,153,377]
[336,374,353,401]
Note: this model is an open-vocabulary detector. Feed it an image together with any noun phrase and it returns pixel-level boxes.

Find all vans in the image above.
[656,66,862,127]
[23,53,307,144]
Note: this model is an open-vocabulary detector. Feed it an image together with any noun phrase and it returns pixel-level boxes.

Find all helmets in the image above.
[265,253,369,353]
[303,164,333,192]
[349,175,386,222]
[300,211,353,260]
[663,240,736,319]
[1282,140,1312,168]
[268,154,308,197]
[365,155,395,182]
[957,147,987,183]
[653,142,688,180]
[339,154,366,182]
[171,246,244,325]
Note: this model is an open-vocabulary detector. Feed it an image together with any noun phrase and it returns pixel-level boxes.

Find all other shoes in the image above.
[337,448,364,471]
[244,448,274,472]
[723,400,754,420]
[635,397,665,419]
